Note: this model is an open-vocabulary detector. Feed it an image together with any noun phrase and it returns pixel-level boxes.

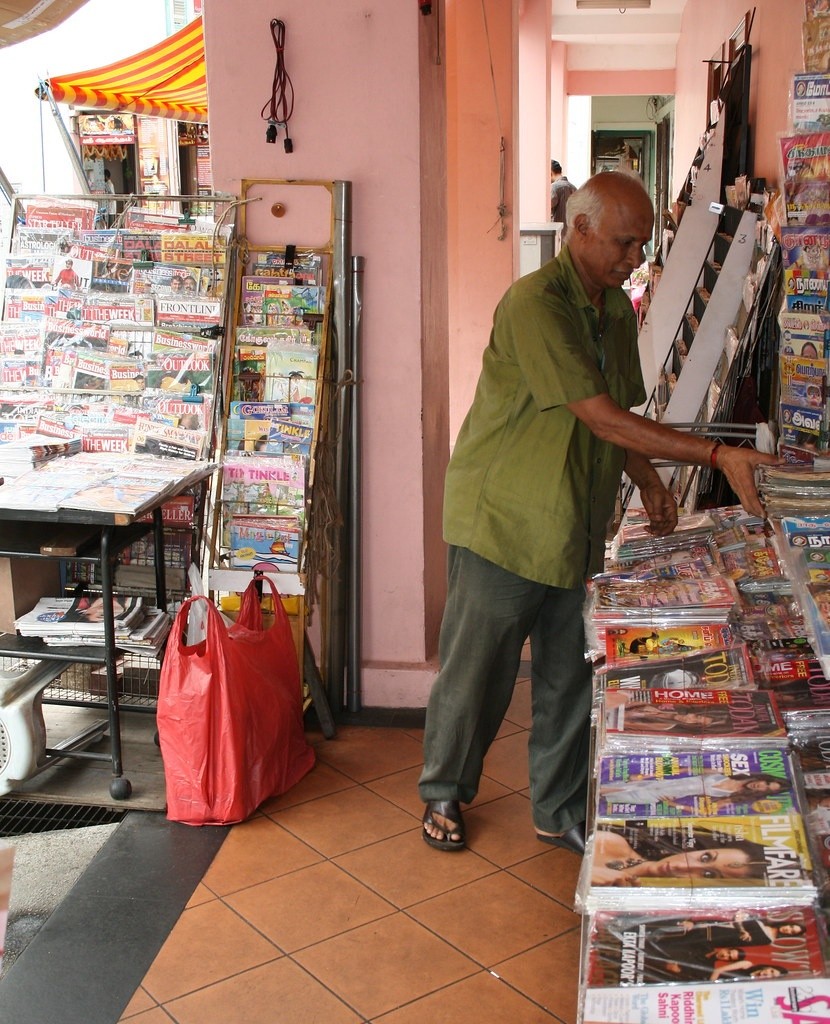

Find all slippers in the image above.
[536,821,585,856]
[422,801,465,851]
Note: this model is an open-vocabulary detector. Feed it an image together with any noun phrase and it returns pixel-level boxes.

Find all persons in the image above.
[169,275,183,292]
[590,824,802,888]
[52,258,81,290]
[598,768,793,815]
[801,342,818,358]
[551,160,578,249]
[414,171,786,854]
[182,276,196,291]
[75,594,132,623]
[608,702,713,731]
[104,169,116,228]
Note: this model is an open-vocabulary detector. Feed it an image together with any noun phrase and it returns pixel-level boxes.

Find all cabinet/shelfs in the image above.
[0,48,829,1024]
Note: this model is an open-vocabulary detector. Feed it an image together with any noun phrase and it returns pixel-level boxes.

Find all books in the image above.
[0,194,324,674]
[574,0,830,1024]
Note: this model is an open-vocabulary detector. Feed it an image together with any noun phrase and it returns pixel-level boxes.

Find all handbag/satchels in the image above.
[156,575,316,826]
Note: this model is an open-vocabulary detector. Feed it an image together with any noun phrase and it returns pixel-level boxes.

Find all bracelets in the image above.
[711,441,724,471]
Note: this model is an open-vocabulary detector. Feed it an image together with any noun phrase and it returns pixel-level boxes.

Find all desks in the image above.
[0,453,214,800]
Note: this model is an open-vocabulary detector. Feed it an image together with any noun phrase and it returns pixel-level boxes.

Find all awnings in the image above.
[35,12,206,194]
